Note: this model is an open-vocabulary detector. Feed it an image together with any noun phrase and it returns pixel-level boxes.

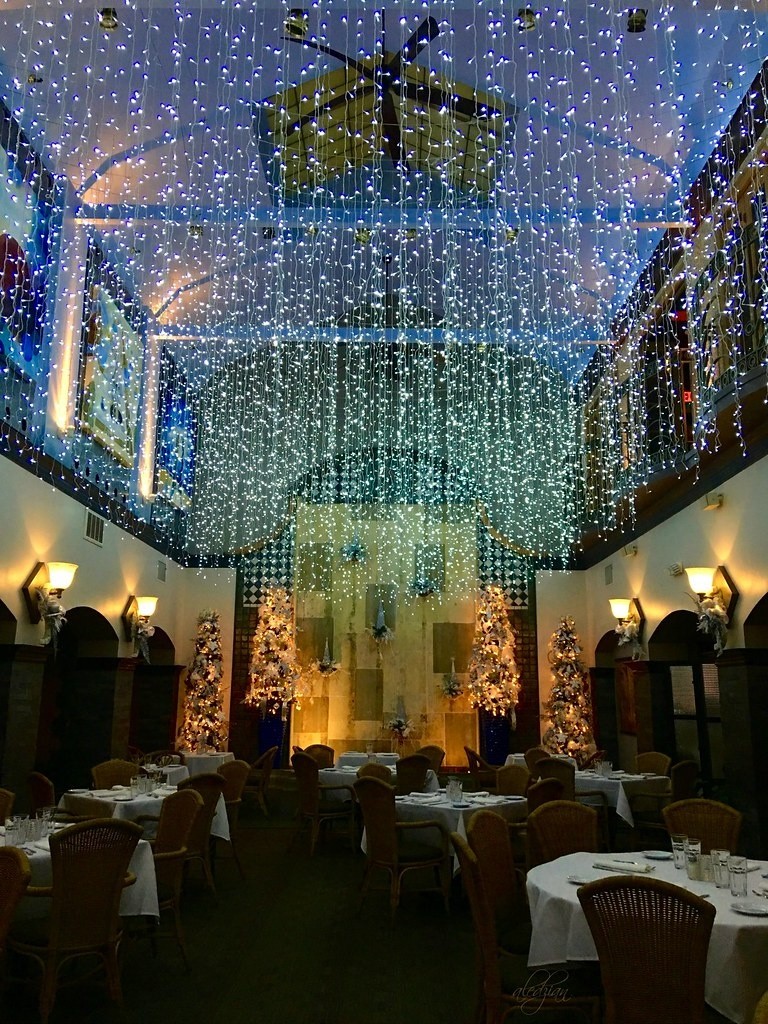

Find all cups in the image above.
[145,754,151,769]
[683,838,701,881]
[727,856,747,897]
[366,743,372,753]
[5,807,54,846]
[698,854,714,882]
[594,759,612,776]
[711,849,730,889]
[130,774,155,797]
[446,780,462,803]
[671,834,688,869]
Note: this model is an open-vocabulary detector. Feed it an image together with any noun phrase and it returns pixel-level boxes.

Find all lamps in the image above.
[609,597,645,662]
[99,7,648,245]
[22,562,79,664]
[121,594,158,665]
[684,566,740,658]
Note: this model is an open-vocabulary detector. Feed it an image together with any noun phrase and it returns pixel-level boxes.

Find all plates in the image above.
[567,873,613,883]
[452,803,471,807]
[730,903,768,914]
[114,797,133,801]
[68,789,88,793]
[643,851,673,859]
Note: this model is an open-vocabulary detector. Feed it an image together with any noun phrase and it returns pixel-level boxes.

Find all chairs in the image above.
[0,744,768,1024]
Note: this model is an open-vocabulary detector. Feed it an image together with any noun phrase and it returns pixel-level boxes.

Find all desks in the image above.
[56,787,230,841]
[525,851,768,1024]
[0,821,160,934]
[360,789,529,899]
[339,752,399,766]
[317,766,440,808]
[572,769,673,853]
[504,753,578,772]
[159,751,235,778]
[92,764,189,786]
